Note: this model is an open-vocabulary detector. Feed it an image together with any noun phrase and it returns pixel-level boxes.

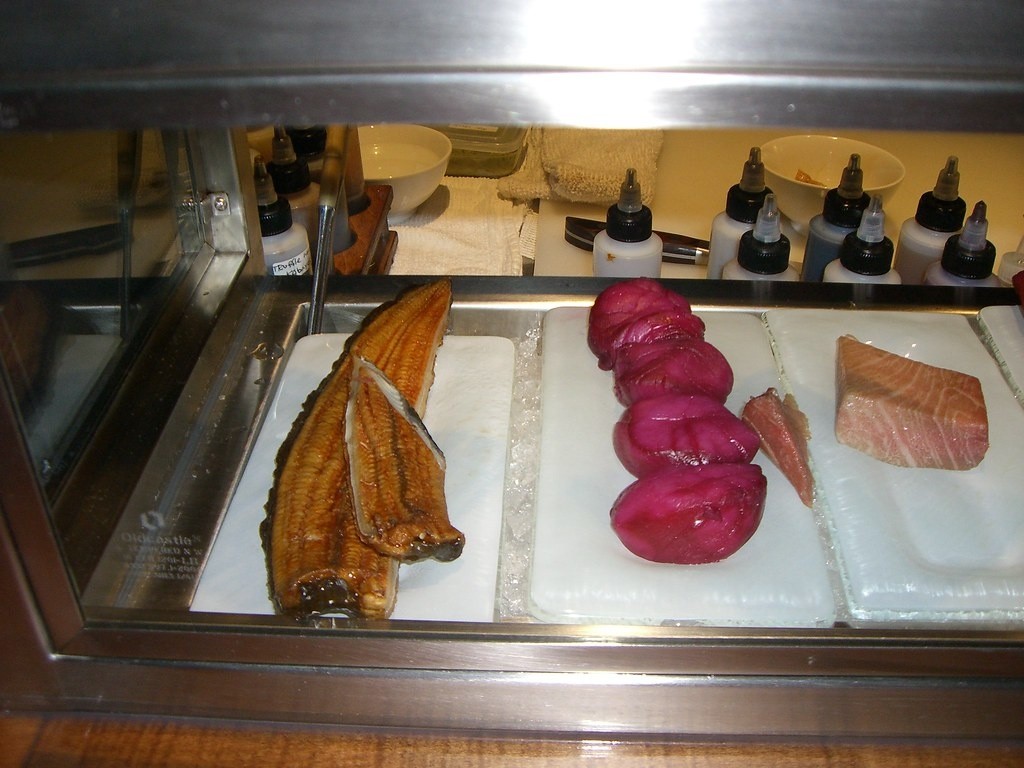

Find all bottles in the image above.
[706,144,1024,281]
[591,168,663,278]
[246,124,367,275]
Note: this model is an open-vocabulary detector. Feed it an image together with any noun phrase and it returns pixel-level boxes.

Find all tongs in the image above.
[564,217,710,266]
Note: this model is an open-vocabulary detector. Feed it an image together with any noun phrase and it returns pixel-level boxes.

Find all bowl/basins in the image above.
[758,134,905,235]
[356,125,453,225]
